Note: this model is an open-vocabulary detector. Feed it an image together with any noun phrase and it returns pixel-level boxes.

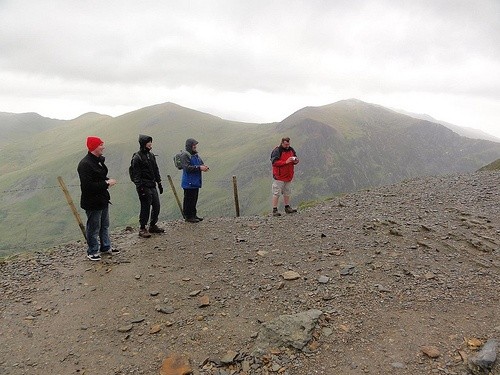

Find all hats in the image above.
[87,137,104,153]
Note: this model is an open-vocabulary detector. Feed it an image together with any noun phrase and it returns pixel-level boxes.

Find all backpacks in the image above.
[173,150,191,170]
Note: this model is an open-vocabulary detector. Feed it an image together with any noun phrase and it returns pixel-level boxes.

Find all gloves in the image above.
[157,183,164,195]
[138,186,146,198]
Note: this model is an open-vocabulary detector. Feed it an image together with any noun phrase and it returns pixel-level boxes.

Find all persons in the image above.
[132,134,165,238]
[180,138,209,223]
[271,137,298,216]
[78,137,120,261]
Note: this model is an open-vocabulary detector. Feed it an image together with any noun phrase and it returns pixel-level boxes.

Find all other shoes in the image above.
[102,247,120,255]
[185,216,199,223]
[139,228,151,238]
[194,215,203,222]
[87,252,102,261]
[149,225,164,233]
[285,206,297,214]
[272,209,281,217]
